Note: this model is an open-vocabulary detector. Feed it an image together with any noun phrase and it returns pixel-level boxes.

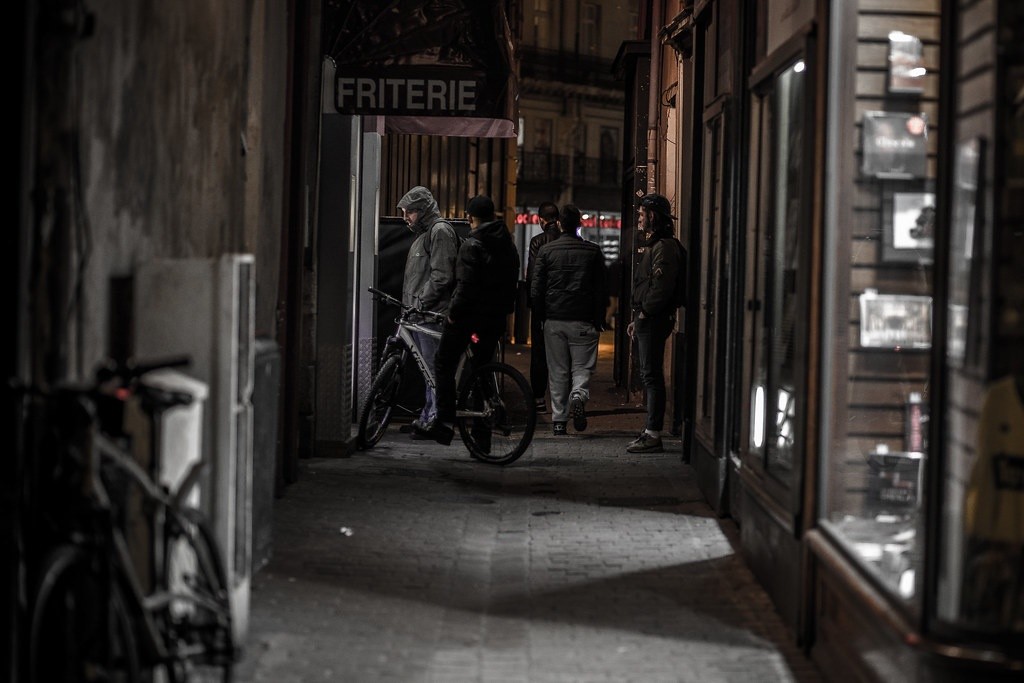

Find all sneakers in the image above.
[626,424,663,452]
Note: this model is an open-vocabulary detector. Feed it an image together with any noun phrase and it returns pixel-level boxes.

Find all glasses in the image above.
[464,209,471,218]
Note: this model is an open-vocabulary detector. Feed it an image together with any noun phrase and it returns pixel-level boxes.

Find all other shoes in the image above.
[507,398,548,414]
[399,419,421,433]
[409,413,455,440]
[571,398,588,431]
[553,422,567,435]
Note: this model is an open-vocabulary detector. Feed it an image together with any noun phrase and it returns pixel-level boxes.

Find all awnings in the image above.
[321,0,521,139]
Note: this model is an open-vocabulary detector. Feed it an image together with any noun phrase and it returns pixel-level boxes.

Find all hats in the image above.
[641,194,678,220]
[466,194,495,217]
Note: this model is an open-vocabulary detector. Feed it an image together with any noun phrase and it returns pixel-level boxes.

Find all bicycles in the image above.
[357,284,538,465]
[0,349,233,683]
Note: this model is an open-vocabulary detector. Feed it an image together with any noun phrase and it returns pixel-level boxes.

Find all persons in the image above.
[413,196,521,459]
[396,185,464,440]
[625,194,689,454]
[527,201,618,436]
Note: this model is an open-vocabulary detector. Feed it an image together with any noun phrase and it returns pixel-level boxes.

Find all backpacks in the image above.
[423,220,489,326]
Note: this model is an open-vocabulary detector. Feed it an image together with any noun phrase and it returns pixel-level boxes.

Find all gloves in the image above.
[439,317,457,336]
